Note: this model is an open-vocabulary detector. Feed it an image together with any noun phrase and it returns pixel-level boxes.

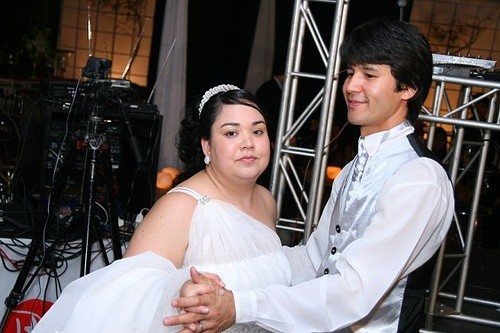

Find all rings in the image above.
[197,321,204,332]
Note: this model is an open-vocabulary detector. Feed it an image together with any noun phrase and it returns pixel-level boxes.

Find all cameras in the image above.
[80,55,112,80]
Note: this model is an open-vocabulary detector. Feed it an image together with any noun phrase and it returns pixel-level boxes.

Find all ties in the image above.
[343,134,371,215]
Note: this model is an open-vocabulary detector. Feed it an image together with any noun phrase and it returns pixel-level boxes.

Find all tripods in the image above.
[4,84,121,310]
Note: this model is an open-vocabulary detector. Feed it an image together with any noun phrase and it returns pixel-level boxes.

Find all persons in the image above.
[164,18,455,332]
[453,91,498,224]
[254,63,296,190]
[420,126,448,166]
[30,84,293,333]
